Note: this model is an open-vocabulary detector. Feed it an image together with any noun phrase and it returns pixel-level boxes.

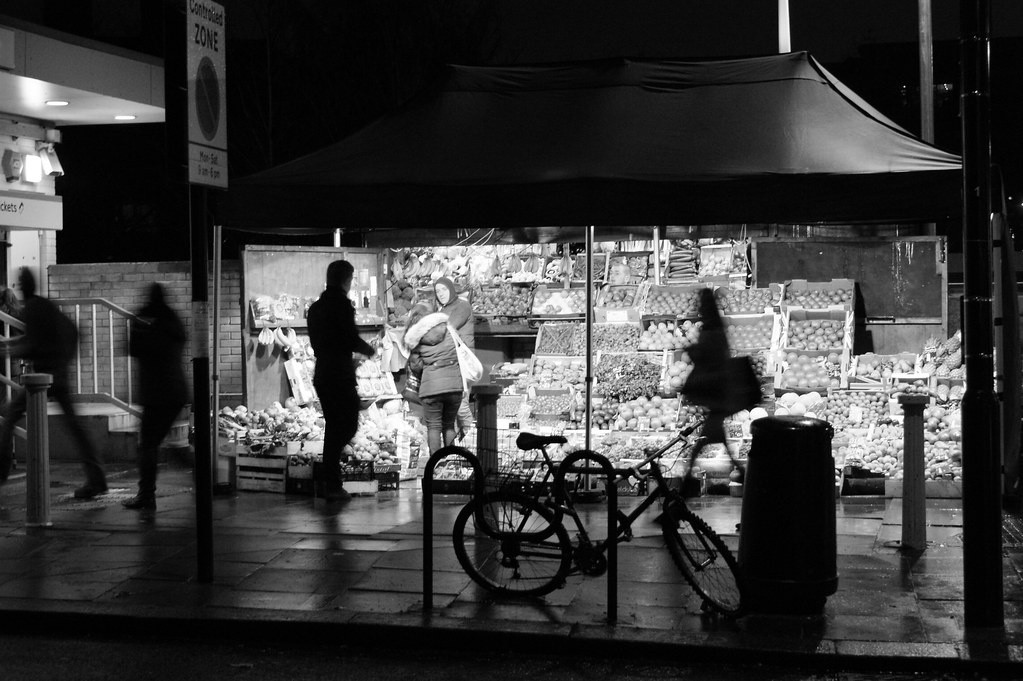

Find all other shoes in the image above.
[122,492,158,509]
[72,480,110,498]
[328,488,353,504]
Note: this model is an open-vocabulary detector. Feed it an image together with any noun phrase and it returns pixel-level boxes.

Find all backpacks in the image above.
[41,294,76,363]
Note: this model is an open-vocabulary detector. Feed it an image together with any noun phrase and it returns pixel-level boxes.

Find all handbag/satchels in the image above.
[447,322,483,388]
[711,354,764,416]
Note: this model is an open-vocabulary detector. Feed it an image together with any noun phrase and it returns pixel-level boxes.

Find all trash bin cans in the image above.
[734,414,840,615]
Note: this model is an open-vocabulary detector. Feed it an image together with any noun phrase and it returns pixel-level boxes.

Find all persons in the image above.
[306,261,376,500]
[679,288,745,487]
[404,279,477,457]
[122,281,189,510]
[0,267,109,498]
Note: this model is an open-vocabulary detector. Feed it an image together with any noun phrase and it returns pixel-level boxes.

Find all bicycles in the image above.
[452,418,751,622]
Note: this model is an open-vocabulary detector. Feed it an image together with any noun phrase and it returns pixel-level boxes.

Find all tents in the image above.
[211,52,1007,505]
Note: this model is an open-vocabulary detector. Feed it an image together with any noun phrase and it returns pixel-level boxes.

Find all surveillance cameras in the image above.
[40,148,66,178]
